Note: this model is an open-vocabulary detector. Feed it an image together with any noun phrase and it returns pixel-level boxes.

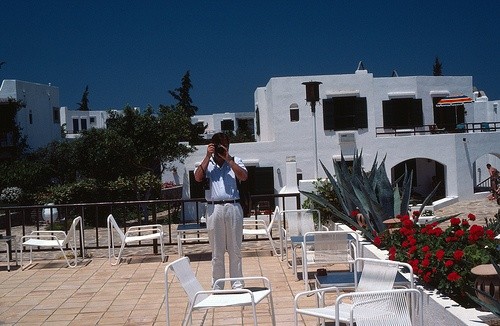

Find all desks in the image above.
[290,233,356,282]
[313,271,411,326]
[0,236,18,271]
[177,224,207,258]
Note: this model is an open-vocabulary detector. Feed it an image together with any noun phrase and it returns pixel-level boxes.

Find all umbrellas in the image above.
[435,92,475,125]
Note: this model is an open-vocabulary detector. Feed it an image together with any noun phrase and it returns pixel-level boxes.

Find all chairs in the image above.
[164,256,276,326]
[244,205,279,256]
[429,123,446,134]
[481,122,490,133]
[107,213,164,265]
[278,209,423,326]
[454,122,466,133]
[20,216,84,271]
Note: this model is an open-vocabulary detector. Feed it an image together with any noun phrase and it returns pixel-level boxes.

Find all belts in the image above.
[207,199,240,204]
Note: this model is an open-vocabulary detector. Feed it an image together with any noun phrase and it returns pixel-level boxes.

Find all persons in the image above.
[193,133,249,291]
[486,164,500,196]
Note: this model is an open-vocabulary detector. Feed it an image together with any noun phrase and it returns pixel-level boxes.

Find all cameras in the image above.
[213,142,225,154]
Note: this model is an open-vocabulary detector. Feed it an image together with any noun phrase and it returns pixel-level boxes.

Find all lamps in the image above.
[277,168,280,175]
